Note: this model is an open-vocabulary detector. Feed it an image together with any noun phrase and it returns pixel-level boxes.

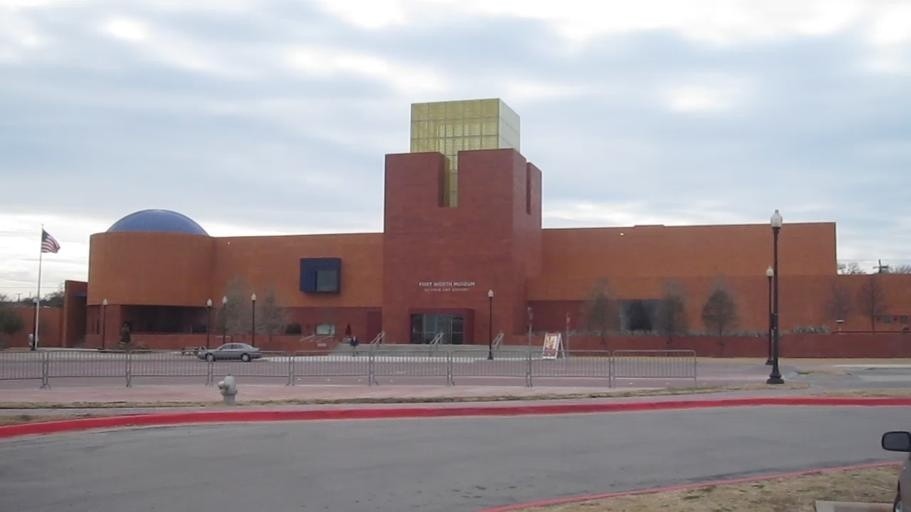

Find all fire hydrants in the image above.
[218,374,239,404]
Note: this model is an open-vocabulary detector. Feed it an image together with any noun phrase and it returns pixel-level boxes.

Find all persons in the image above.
[349,334,360,357]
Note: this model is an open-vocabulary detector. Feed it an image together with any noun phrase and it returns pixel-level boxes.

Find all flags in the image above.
[41,228,61,255]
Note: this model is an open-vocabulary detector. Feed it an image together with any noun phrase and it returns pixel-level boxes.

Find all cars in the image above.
[881,429,911,511]
[198,342,263,363]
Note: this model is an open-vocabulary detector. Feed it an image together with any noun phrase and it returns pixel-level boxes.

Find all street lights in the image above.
[222,296,228,344]
[251,292,258,345]
[768,209,785,384]
[527,305,535,386]
[102,298,109,349]
[32,297,38,351]
[487,288,495,360]
[207,297,214,349]
[765,266,775,364]
